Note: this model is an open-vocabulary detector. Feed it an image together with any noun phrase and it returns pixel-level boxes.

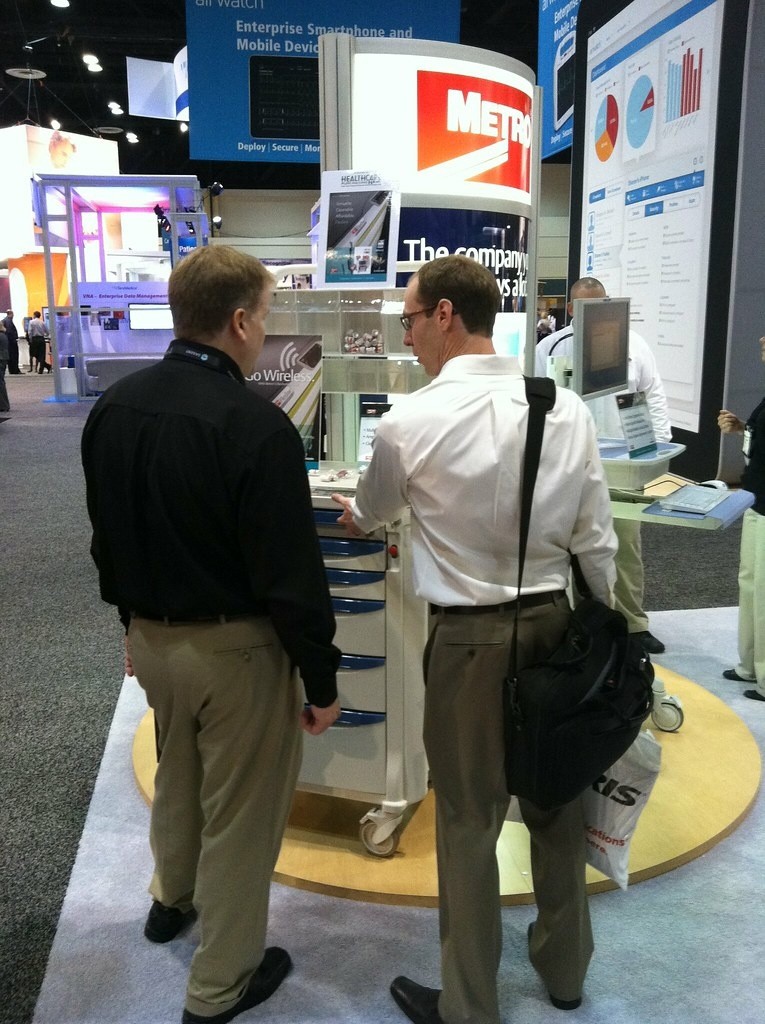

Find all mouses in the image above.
[697,480,728,492]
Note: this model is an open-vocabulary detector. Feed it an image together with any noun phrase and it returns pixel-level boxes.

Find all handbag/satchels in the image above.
[504,597,656,810]
[582,731,661,889]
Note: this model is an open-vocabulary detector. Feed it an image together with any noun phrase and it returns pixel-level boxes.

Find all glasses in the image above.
[400,304,458,330]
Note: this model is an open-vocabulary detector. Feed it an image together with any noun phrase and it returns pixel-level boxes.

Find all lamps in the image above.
[201,181,223,200]
[208,216,223,230]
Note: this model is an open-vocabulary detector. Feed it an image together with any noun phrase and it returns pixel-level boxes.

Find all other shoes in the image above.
[744,690,765,701]
[723,670,756,682]
[628,631,665,652]
[47,364,52,373]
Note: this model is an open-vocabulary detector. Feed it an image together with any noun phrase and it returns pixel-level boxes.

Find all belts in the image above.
[136,613,245,623]
[442,590,565,614]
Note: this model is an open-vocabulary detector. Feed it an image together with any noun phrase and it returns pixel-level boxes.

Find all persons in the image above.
[27,311,51,374]
[535,277,672,653]
[718,337,765,702]
[2,309,24,374]
[0,321,10,415]
[537,312,551,327]
[80,245,343,1024]
[48,130,76,168]
[331,255,619,1024]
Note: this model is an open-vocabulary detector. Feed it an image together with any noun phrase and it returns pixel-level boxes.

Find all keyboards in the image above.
[660,485,732,514]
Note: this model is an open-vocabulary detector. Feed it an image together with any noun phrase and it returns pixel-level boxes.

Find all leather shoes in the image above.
[144,901,199,943]
[391,976,444,1024]
[182,947,290,1024]
[527,921,582,1009]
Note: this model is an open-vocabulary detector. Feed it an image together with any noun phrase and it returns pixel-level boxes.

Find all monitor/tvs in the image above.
[572,298,628,402]
